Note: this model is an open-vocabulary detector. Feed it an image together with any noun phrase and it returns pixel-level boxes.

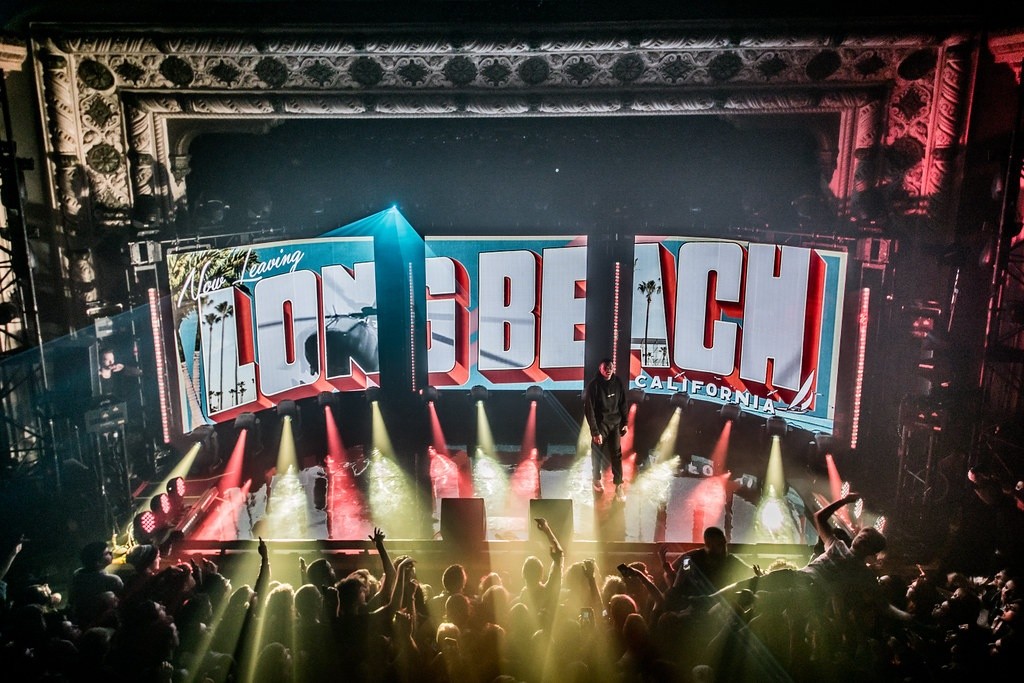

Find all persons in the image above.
[1,492,1024,683]
[99,348,144,397]
[585,358,629,502]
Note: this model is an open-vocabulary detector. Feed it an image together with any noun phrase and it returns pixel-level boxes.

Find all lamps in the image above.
[150,493,176,528]
[133,511,159,546]
[167,477,192,517]
[189,384,837,479]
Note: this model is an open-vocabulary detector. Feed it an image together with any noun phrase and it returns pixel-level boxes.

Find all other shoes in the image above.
[615,484,627,503]
[592,480,604,493]
